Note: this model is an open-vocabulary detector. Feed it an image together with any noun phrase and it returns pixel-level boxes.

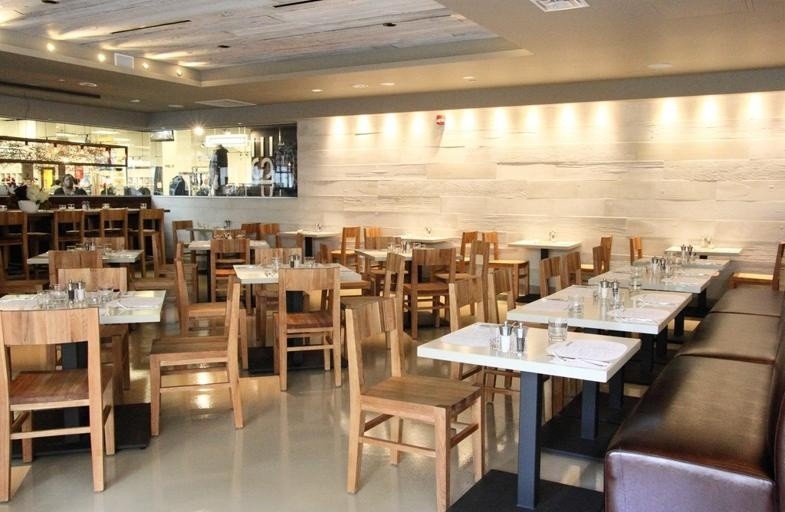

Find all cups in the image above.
[629,265,644,291]
[547,317,568,344]
[568,293,585,313]
[387,242,426,257]
[56,200,148,209]
[667,250,683,273]
[67,243,113,258]
[304,256,316,269]
[40,284,113,311]
[272,257,282,274]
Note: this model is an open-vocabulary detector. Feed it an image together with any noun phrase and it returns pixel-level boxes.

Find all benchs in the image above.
[602,281,785,511]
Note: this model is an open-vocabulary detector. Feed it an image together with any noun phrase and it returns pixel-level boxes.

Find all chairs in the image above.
[731,238,785,291]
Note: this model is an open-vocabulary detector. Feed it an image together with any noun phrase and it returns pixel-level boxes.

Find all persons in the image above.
[52,173,186,196]
[206,142,229,196]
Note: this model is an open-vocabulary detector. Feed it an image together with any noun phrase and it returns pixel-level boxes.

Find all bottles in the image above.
[681,244,697,264]
[401,240,410,253]
[85,238,96,252]
[67,279,85,304]
[498,320,527,354]
[701,236,711,248]
[288,254,301,267]
[651,256,666,274]
[598,276,622,307]
